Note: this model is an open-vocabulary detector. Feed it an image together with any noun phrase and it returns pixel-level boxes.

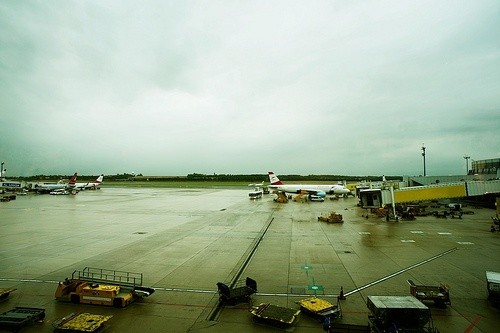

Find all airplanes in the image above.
[247,171,350,198]
[34,172,105,192]
